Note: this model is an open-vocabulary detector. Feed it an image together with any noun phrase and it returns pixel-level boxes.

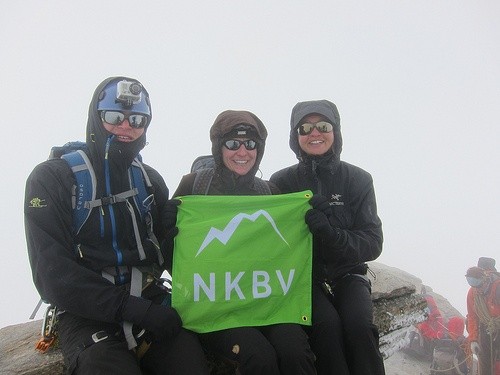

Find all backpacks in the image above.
[190,155,271,196]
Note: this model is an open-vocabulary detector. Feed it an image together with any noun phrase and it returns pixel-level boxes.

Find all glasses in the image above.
[99,110,148,129]
[223,140,259,150]
[296,122,334,136]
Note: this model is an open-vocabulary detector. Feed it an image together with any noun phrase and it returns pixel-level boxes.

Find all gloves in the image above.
[470,342,481,355]
[122,294,182,341]
[304,210,336,243]
[156,198,182,241]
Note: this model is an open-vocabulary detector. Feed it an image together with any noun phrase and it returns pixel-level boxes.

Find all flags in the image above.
[173,190,313,334]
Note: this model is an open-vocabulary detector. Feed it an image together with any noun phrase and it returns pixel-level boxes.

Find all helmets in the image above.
[97,78,151,117]
[220,124,258,137]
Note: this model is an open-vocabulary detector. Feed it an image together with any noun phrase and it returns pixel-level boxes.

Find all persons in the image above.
[270,99,385,375]
[162,110,317,375]
[23,77,211,375]
[465,266,500,375]
